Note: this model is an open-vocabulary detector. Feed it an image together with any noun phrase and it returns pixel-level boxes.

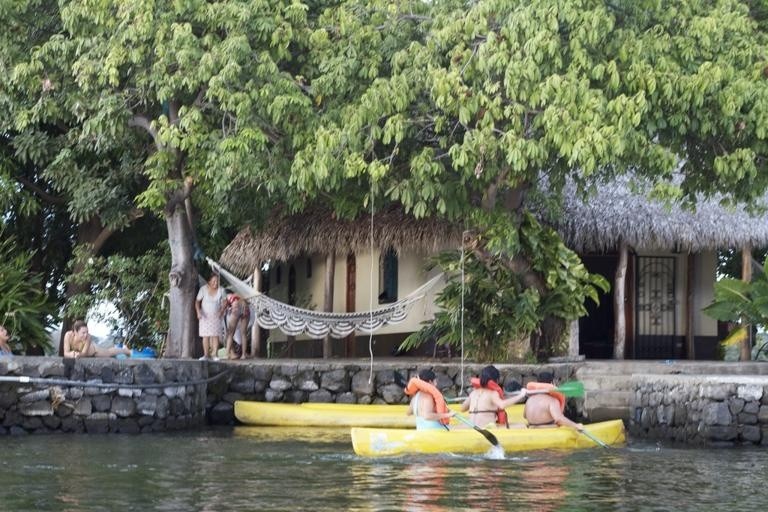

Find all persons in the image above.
[63,320,131,359]
[403,368,457,430]
[459,366,527,430]
[223,288,250,360]
[0,325,14,356]
[523,371,586,432]
[194,273,225,362]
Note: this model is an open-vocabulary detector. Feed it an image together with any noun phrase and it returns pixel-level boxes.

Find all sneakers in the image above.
[198,355,220,361]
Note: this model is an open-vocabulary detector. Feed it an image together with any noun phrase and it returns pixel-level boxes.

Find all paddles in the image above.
[448,409,501,447]
[574,429,632,456]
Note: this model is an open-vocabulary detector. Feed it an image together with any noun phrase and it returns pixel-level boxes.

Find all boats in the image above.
[233,399,526,427]
[350,419,627,457]
